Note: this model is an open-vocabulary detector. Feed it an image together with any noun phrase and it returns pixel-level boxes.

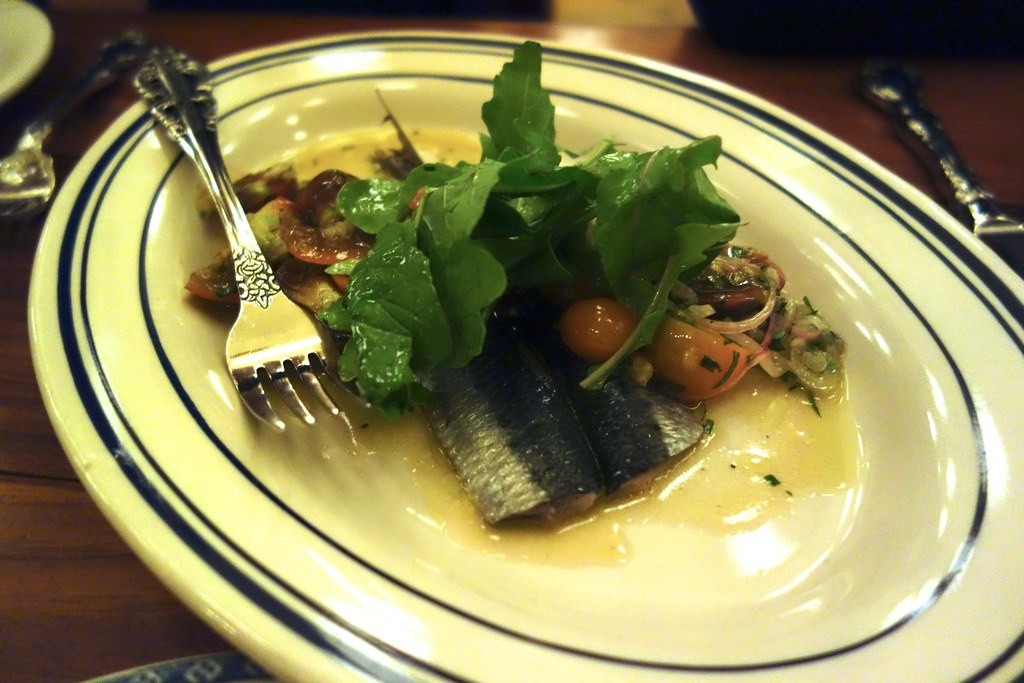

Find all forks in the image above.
[132,44,372,431]
[0,28,139,224]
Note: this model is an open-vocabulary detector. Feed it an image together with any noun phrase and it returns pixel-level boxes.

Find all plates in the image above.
[27,29,1024,683]
[0,0,55,104]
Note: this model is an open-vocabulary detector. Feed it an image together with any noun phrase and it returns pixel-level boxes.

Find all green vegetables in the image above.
[321,38,742,417]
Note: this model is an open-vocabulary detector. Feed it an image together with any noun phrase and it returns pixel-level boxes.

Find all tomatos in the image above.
[561,296,639,360]
[644,315,748,402]
